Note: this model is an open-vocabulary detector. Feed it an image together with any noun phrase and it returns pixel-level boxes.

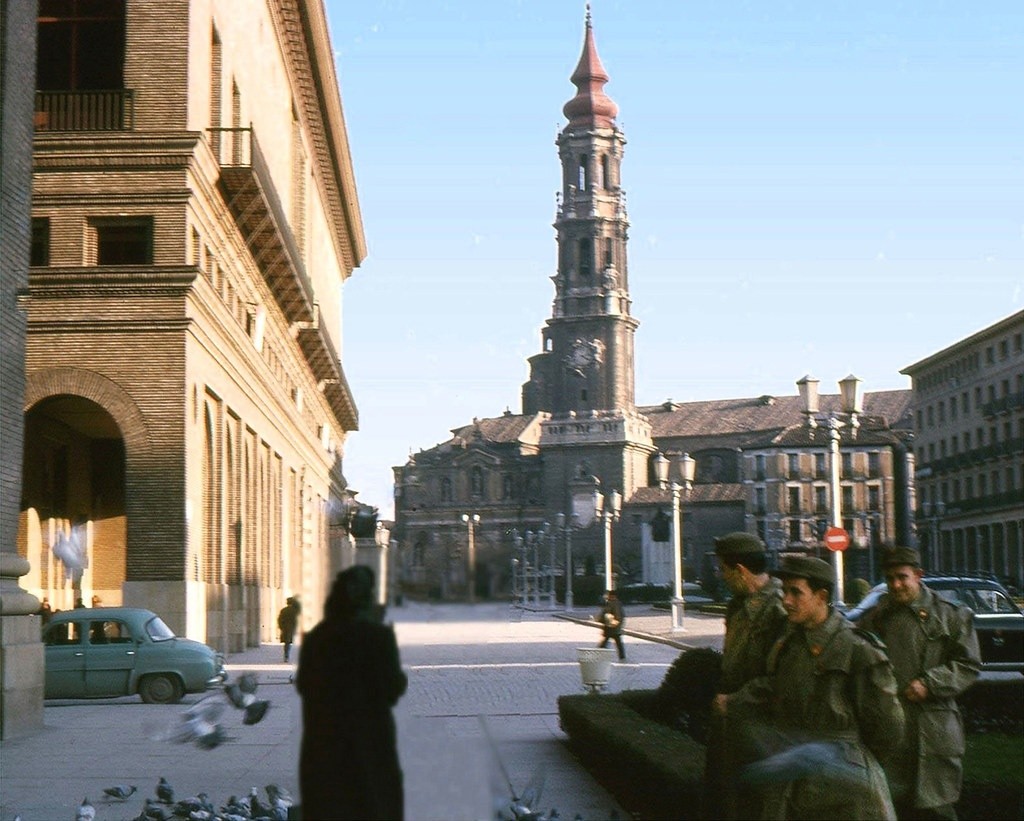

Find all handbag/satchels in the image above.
[604,613,620,628]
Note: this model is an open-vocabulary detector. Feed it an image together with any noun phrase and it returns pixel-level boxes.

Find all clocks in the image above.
[575,346,595,367]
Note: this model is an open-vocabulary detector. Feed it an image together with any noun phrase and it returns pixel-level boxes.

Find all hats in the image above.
[704,532,766,554]
[883,544,922,567]
[770,552,836,584]
[843,577,872,605]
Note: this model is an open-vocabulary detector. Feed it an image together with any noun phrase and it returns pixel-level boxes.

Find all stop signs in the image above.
[824,527,850,552]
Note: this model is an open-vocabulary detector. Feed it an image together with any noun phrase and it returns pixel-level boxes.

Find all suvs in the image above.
[837,569,1024,664]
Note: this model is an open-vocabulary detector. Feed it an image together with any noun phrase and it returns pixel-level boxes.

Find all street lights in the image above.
[461,514,480,602]
[796,378,864,601]
[592,488,622,592]
[511,512,579,616]
[654,451,696,627]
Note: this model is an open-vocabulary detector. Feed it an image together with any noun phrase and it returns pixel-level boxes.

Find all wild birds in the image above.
[101,785,137,802]
[14,815,21,821]
[77,796,97,821]
[142,776,293,821]
[509,797,584,821]
[142,670,272,751]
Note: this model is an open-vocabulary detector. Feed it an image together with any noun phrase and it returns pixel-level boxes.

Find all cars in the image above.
[41,607,229,705]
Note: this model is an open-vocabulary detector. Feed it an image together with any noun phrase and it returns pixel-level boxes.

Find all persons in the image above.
[278,597,300,662]
[296,565,409,821]
[42,595,51,611]
[703,534,981,821]
[596,590,625,660]
[74,595,104,629]
